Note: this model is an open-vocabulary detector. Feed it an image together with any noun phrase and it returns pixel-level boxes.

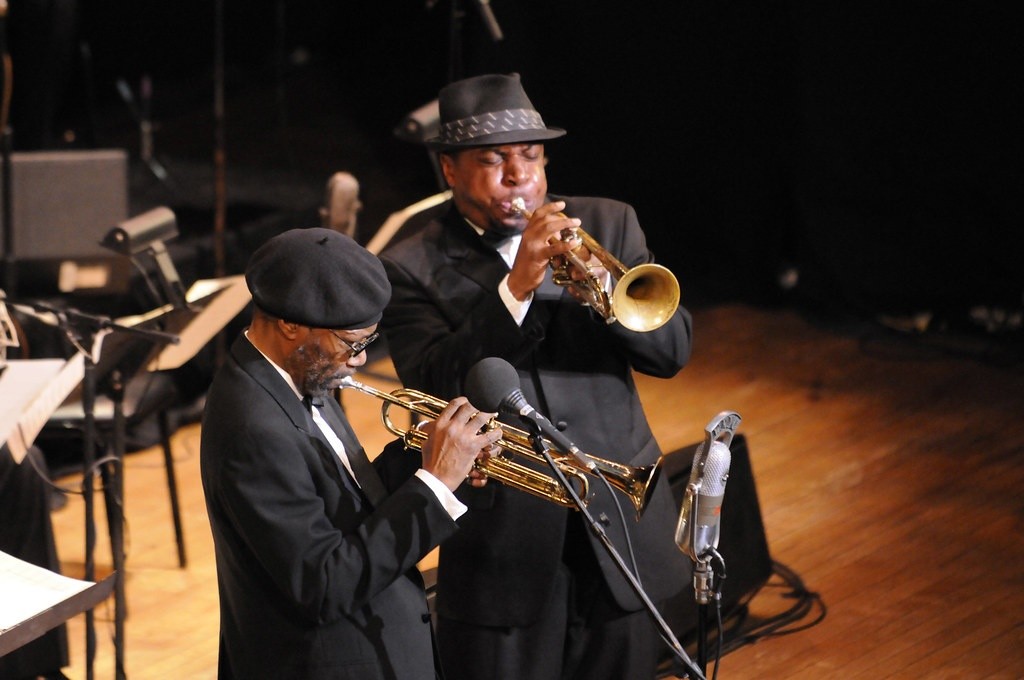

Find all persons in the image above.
[0,440,71,680]
[199,227,505,679]
[379,71,694,679]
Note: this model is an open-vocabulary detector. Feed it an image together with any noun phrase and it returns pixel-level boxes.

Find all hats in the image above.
[245,228,392,330]
[422,72,567,148]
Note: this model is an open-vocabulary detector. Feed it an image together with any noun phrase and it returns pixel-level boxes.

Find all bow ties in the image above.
[302,395,324,413]
[482,229,507,247]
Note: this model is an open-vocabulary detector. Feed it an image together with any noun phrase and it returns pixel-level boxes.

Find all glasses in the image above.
[329,329,379,357]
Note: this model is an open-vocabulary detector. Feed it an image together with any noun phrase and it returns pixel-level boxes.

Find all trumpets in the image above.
[339,373,667,523]
[509,195,682,333]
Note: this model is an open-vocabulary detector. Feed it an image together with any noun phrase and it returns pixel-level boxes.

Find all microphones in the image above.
[674,440,731,606]
[466,356,602,475]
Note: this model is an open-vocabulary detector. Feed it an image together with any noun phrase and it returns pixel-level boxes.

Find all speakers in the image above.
[661,434,775,626]
